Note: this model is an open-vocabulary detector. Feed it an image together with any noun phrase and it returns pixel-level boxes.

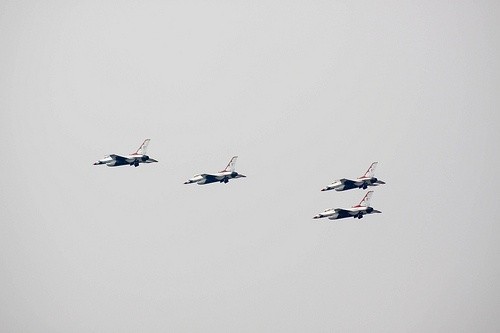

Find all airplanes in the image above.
[320,162,386,192]
[312,191,382,220]
[184,155,247,185]
[92,139,158,167]
